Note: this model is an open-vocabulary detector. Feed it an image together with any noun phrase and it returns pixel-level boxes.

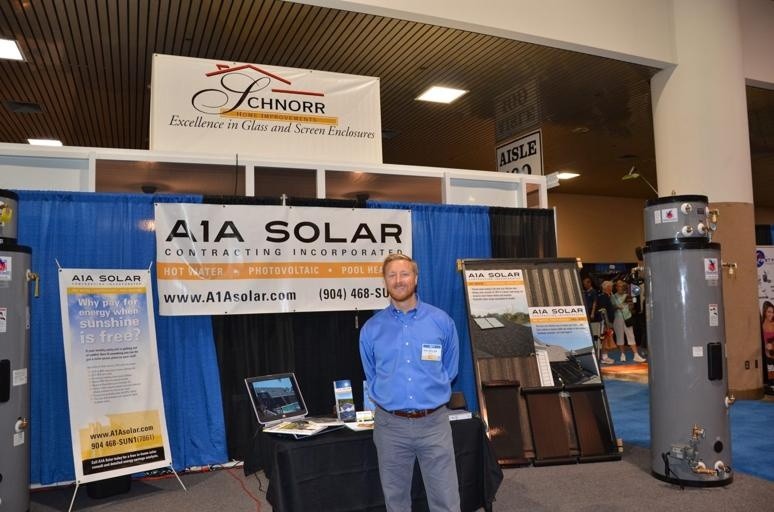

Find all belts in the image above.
[376,402,445,420]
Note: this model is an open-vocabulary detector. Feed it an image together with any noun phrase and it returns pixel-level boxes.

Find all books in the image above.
[332,379,355,419]
[344,421,374,431]
[305,417,345,426]
[448,409,472,422]
[261,420,328,435]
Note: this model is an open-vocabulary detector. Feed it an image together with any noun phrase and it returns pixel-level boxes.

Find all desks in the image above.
[246,412,485,512]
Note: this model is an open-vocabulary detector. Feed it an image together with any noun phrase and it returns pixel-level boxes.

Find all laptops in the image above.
[244,372,345,440]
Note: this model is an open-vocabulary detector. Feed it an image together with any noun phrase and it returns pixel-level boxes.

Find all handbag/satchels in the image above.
[624,316,636,328]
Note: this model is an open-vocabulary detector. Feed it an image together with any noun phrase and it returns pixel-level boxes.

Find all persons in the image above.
[356,252,462,511]
[762,300,774,360]
[595,281,617,364]
[609,278,647,362]
[582,278,601,357]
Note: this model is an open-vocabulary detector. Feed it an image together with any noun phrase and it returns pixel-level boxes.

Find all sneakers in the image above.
[620,353,627,362]
[600,357,615,364]
[633,355,647,363]
